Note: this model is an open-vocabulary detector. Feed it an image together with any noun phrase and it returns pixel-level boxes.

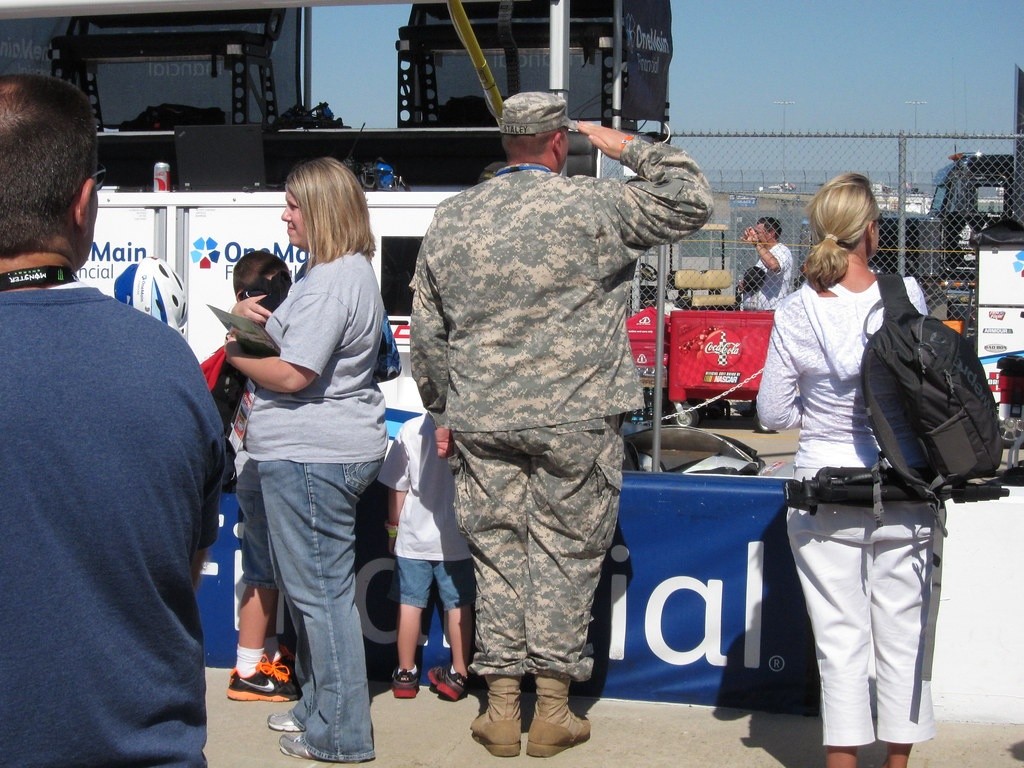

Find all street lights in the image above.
[905,101,927,169]
[773,101,795,169]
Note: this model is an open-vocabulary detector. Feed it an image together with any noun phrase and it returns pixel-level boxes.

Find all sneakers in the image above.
[226,654,303,702]
[271,646,296,672]
[267,712,301,731]
[279,733,322,760]
[428,663,468,701]
[391,666,420,698]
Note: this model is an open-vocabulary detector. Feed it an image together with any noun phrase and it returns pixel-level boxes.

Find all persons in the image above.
[198,249,303,701]
[408,92,712,758]
[743,217,793,312]
[374,411,475,701]
[0,72,225,768]
[225,156,390,764]
[757,173,940,768]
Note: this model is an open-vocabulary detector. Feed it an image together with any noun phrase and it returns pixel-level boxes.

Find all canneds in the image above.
[154,162,170,193]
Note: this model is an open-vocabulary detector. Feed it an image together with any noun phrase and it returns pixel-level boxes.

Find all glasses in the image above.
[79,163,106,192]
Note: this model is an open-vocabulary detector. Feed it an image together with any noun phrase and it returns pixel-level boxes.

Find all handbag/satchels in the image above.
[373,310,402,383]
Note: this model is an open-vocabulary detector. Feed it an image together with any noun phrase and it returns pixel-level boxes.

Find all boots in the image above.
[526,676,590,757]
[470,674,522,757]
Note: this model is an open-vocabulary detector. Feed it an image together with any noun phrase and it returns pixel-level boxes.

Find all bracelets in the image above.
[621,136,634,149]
[752,240,762,247]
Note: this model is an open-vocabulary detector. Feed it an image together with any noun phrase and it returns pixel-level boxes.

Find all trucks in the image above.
[623,154,1024,449]
[71,126,505,464]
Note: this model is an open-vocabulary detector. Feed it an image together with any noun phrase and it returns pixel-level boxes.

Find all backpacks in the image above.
[861,273,1003,500]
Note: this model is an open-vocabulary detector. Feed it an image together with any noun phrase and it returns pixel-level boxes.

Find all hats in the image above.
[500,92,576,134]
